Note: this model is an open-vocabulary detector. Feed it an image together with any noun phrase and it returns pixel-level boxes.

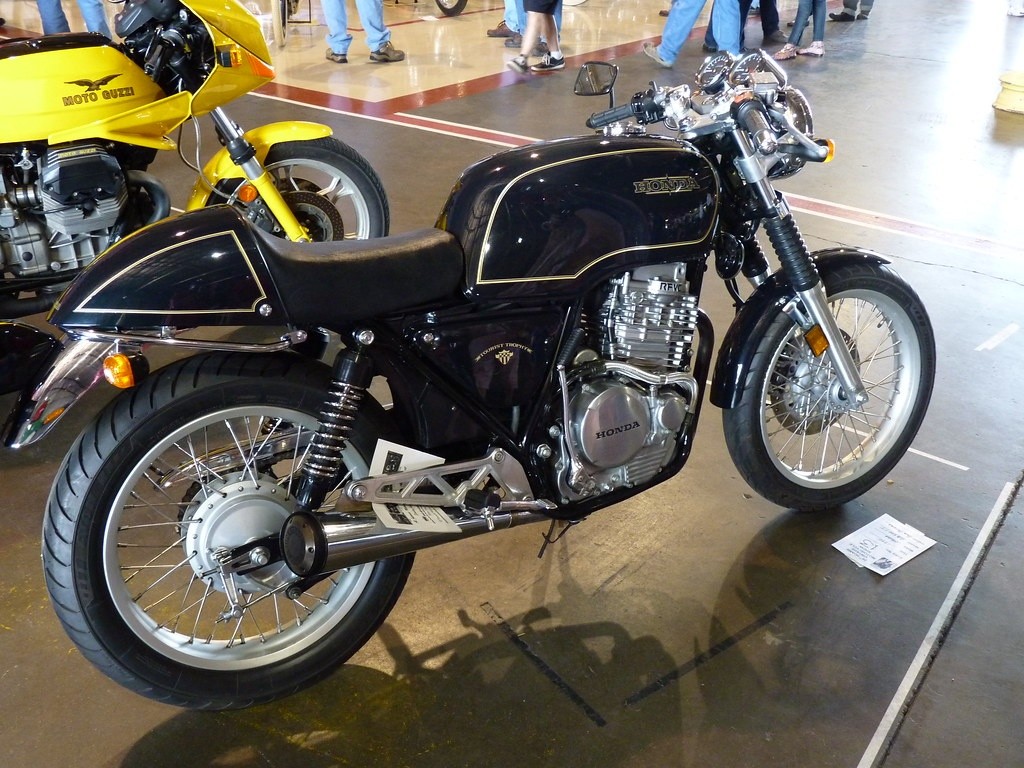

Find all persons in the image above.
[320,0,405,63]
[486,0,565,74]
[643,0,876,70]
[35,0,111,40]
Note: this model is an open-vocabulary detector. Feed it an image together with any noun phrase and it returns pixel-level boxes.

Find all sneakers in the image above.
[797,41,825,56]
[504,33,523,48]
[642,42,673,68]
[507,54,528,74]
[370,40,405,62]
[775,46,797,59]
[486,20,518,37]
[326,48,348,63]
[530,53,565,71]
[530,42,551,56]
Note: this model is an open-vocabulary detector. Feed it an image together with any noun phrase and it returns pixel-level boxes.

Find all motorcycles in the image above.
[0,0,391,318]
[0,44,938,716]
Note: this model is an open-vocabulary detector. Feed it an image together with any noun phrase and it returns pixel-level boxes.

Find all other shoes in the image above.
[787,18,810,27]
[857,9,870,19]
[1007,5,1024,16]
[829,11,855,21]
[765,29,790,43]
[740,48,748,52]
[702,43,718,53]
